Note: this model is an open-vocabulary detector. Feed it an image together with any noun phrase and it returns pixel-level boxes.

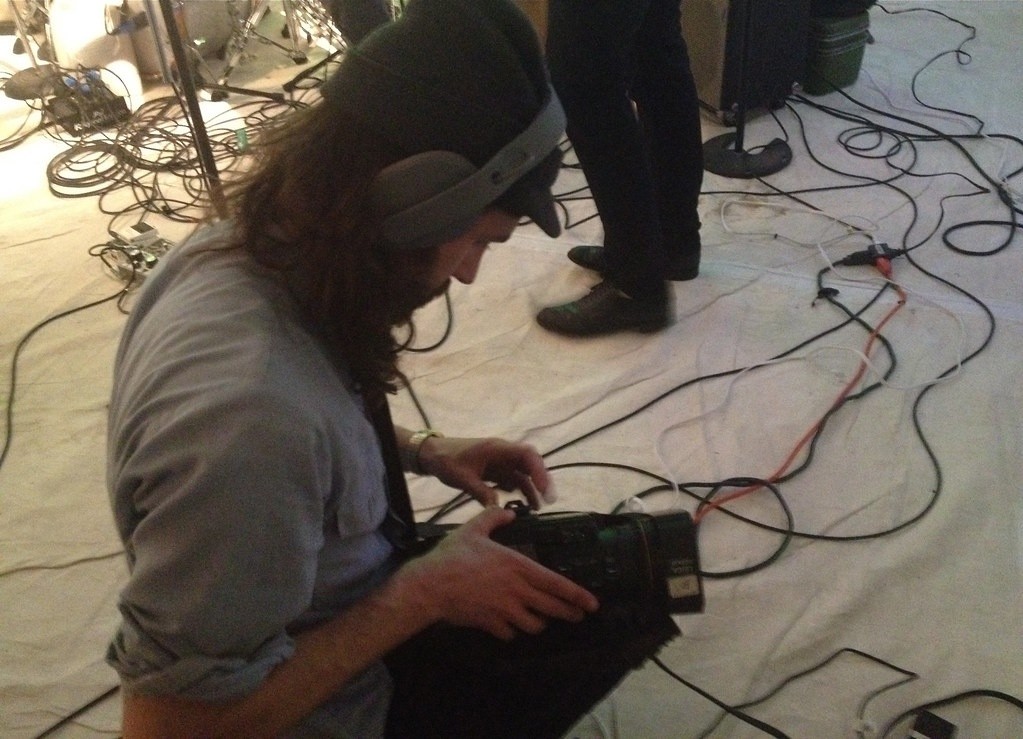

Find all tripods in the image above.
[129,0,348,147]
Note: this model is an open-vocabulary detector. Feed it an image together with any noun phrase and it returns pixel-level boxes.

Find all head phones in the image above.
[372,79,567,255]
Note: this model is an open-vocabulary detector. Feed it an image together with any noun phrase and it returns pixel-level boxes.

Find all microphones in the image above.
[111,12,149,38]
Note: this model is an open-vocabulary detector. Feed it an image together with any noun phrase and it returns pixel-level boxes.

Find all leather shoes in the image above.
[567,245,700,282]
[536,279,677,338]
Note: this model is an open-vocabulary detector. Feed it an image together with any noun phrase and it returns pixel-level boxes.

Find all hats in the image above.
[319,0,566,238]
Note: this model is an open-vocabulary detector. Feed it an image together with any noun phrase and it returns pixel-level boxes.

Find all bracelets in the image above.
[407,430,445,477]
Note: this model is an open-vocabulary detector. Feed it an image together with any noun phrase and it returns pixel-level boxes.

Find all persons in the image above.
[538,0,702,335]
[104,0,681,739]
[321,0,396,51]
